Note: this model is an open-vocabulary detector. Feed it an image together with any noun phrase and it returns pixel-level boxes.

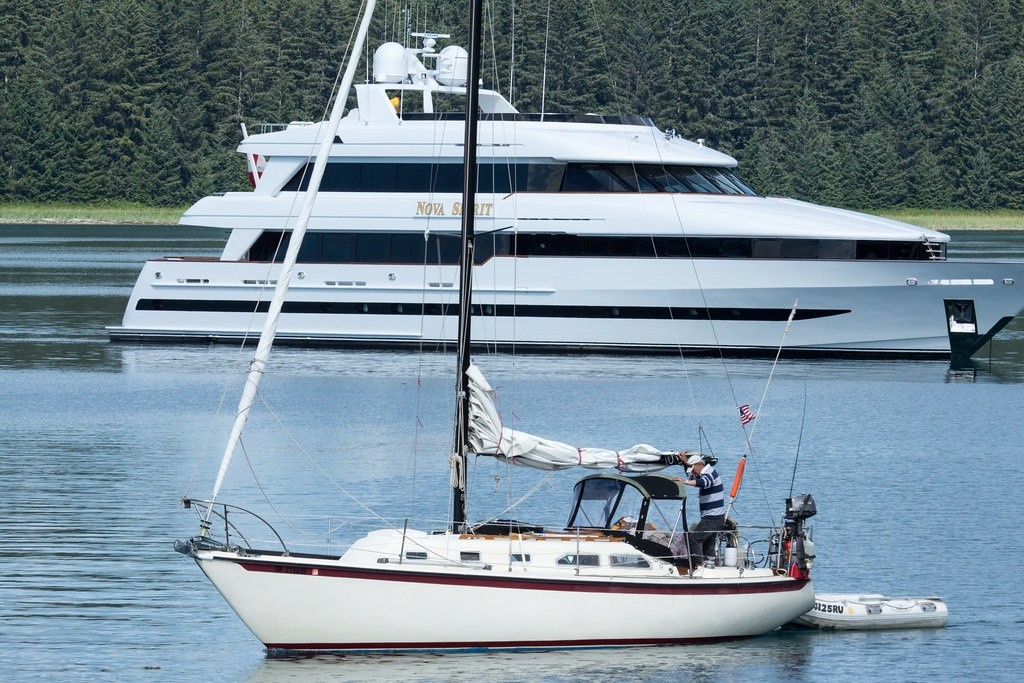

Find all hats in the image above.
[685,455,706,474]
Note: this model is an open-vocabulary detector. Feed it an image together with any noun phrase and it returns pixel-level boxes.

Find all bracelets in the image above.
[683,480,688,485]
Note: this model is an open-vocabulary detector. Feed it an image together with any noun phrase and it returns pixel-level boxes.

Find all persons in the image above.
[389,94,402,118]
[672,449,726,573]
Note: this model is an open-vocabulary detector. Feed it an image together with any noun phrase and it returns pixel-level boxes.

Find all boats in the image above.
[105,29,1024,363]
[781,592,950,629]
[177,1,818,657]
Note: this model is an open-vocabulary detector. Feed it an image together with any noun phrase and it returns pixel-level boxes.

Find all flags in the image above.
[246,154,271,188]
[738,403,757,426]
